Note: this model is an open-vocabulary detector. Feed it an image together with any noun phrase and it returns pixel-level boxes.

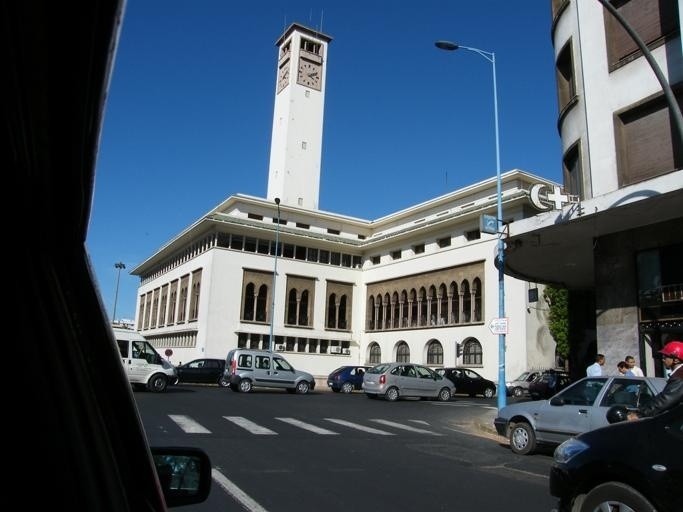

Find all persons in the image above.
[626,341,683,420]
[133,344,144,358]
[616,361,637,378]
[585,354,605,377]
[542,367,557,400]
[619,356,646,377]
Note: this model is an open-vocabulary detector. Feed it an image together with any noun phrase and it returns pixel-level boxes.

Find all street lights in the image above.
[431,38,509,412]
[267,196,281,352]
[111,260,126,322]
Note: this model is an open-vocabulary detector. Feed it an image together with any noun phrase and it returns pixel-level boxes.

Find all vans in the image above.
[109,328,178,392]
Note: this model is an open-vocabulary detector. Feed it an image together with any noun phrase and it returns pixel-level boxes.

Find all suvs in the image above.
[503,368,541,398]
[222,347,316,394]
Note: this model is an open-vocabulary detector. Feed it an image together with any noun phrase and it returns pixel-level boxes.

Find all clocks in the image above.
[296,56,323,92]
[276,57,291,95]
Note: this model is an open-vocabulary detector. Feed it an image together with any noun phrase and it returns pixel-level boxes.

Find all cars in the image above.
[493,375,671,458]
[361,363,457,402]
[2,5,213,511]
[433,368,495,400]
[327,365,373,393]
[527,368,570,399]
[546,403,681,512]
[172,357,231,389]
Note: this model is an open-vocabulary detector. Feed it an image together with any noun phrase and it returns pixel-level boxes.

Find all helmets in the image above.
[656,341,683,361]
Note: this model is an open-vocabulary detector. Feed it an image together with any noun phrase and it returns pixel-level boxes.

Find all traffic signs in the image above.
[485,317,510,336]
